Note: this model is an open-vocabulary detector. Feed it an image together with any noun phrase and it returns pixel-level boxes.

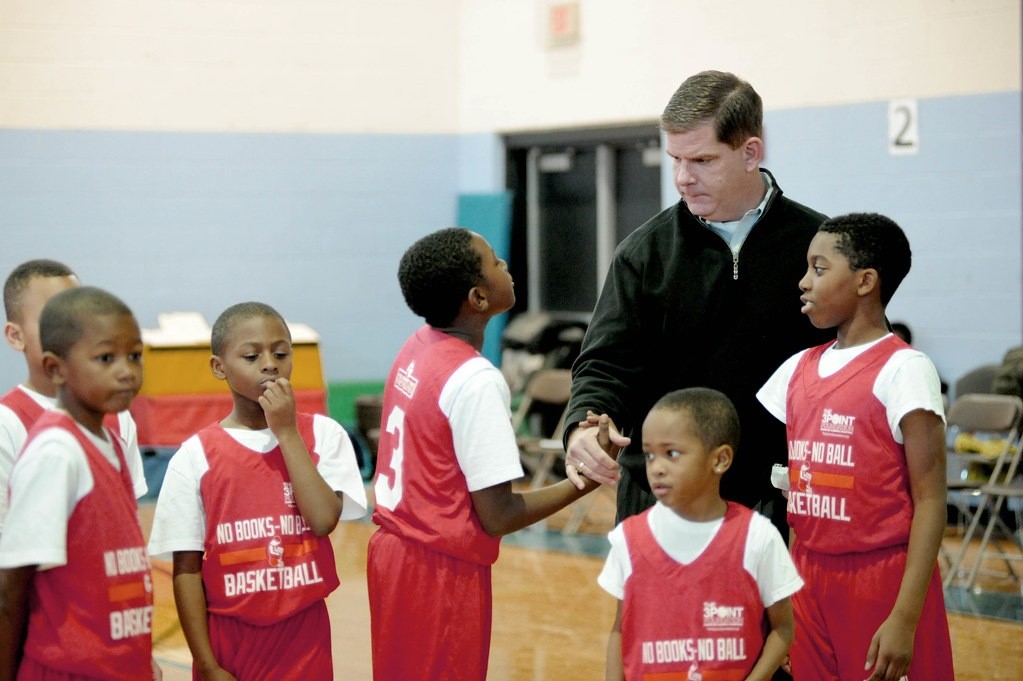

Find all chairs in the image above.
[506,369,620,544]
[942,393,1023,590]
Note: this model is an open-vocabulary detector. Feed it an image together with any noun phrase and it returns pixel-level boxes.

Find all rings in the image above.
[576,463,585,471]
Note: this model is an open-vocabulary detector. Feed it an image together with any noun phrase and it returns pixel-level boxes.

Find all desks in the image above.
[133,322,329,463]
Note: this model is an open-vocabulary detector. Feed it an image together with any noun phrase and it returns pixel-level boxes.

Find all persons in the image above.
[0,258,163,681]
[148,302,368,681]
[561,76,955,681]
[366,227,618,681]
